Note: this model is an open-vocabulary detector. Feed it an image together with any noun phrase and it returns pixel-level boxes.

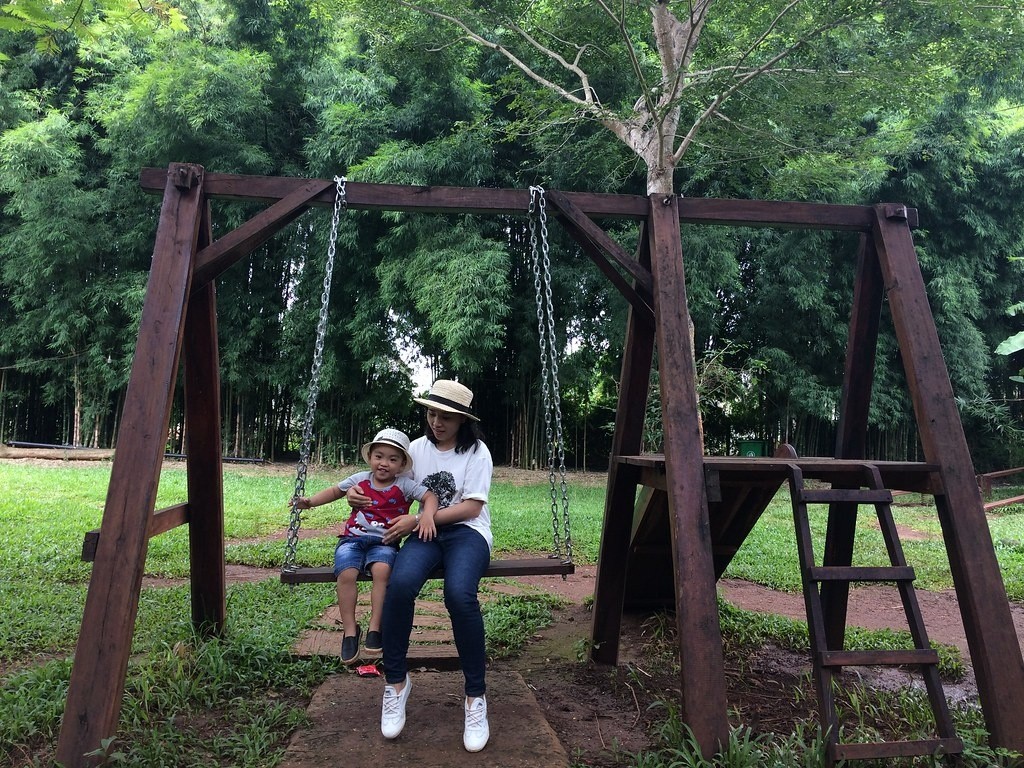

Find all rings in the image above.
[394,531,401,538]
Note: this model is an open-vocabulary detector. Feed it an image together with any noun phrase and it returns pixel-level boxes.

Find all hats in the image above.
[414,379,481,422]
[361,429,414,474]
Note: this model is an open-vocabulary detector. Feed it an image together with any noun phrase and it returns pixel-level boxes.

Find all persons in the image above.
[288,429,439,664]
[346,380,492,753]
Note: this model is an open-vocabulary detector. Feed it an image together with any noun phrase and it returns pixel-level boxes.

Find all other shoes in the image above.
[364,624,383,652]
[340,624,363,665]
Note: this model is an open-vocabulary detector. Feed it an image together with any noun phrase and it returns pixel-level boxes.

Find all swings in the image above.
[277,176,576,583]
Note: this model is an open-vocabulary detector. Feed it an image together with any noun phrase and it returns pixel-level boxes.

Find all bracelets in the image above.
[415,516,420,525]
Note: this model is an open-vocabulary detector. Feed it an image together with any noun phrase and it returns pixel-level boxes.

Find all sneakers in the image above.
[381,672,412,738]
[463,693,489,753]
